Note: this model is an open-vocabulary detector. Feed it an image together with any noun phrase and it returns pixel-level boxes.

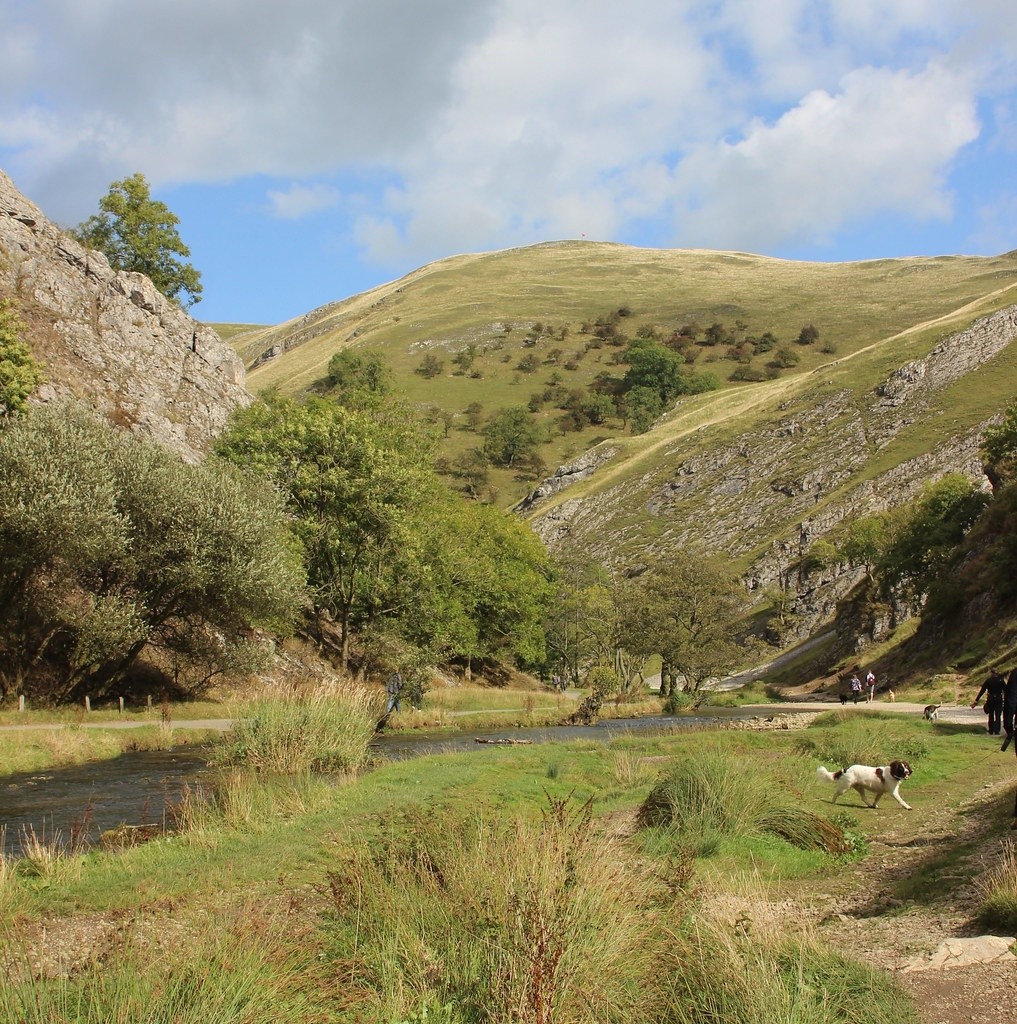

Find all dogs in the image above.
[923,705,941,722]
[816,760,913,810]
[839,693,849,705]
[888,689,895,703]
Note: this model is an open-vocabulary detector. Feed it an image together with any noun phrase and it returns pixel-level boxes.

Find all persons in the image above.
[1003,667,1017,759]
[970,668,1007,735]
[386,667,402,713]
[865,669,875,703]
[552,672,568,691]
[849,674,862,704]
[1002,671,1011,685]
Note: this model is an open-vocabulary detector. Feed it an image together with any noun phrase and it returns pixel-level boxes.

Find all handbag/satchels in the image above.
[983,693,994,715]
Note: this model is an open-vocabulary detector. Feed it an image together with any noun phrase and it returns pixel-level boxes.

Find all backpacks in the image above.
[868,675,873,685]
